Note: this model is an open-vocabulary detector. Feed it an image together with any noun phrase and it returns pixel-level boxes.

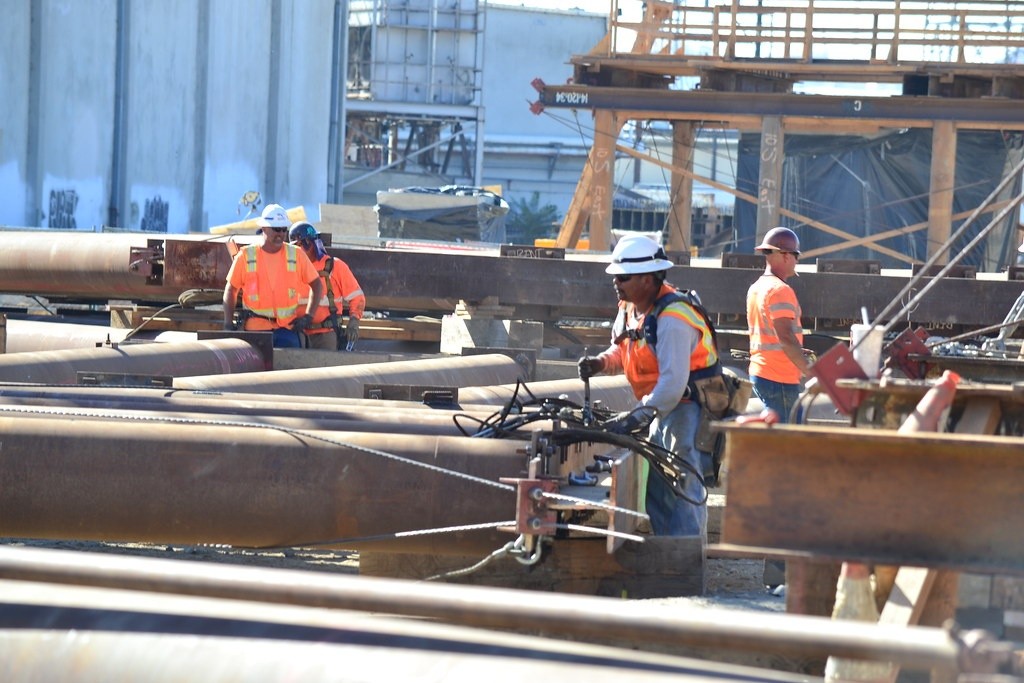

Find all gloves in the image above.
[343,316,359,340]
[605,411,638,436]
[289,314,313,333]
[578,357,602,380]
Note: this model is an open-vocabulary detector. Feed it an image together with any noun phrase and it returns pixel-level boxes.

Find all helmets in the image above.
[257,204,292,227]
[288,221,318,242]
[753,226,799,254]
[605,233,674,275]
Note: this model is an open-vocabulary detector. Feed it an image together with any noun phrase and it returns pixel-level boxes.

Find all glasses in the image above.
[614,273,641,282]
[269,226,287,232]
[788,251,799,258]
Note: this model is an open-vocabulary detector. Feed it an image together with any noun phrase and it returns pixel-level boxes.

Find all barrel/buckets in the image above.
[852,322,884,379]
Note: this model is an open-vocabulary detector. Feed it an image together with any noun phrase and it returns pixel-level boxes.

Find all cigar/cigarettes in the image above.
[793,270,800,277]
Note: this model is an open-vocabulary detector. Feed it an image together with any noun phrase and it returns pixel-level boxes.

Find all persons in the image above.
[579,234,722,555]
[745,228,812,425]
[286,223,365,352]
[222,203,322,348]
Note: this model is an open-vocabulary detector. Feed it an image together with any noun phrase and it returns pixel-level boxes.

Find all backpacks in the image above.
[643,291,753,467]
